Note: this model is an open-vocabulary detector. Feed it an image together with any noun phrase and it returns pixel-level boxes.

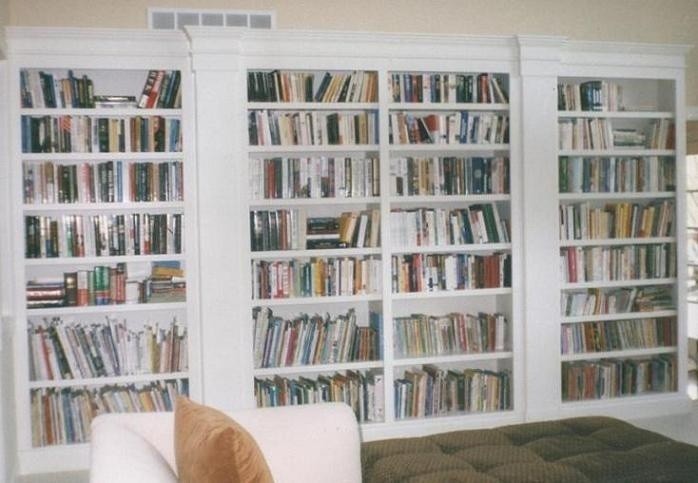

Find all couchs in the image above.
[88,401,366,482]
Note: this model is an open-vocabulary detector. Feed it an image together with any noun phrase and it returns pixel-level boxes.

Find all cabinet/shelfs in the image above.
[2,26,209,475]
[383,27,526,430]
[213,25,387,445]
[536,46,681,410]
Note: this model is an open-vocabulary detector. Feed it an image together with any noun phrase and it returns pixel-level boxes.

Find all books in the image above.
[555,81,681,404]
[245,64,512,423]
[19,68,188,447]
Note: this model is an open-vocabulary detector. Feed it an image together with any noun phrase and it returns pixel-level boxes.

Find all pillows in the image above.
[168,392,272,482]
